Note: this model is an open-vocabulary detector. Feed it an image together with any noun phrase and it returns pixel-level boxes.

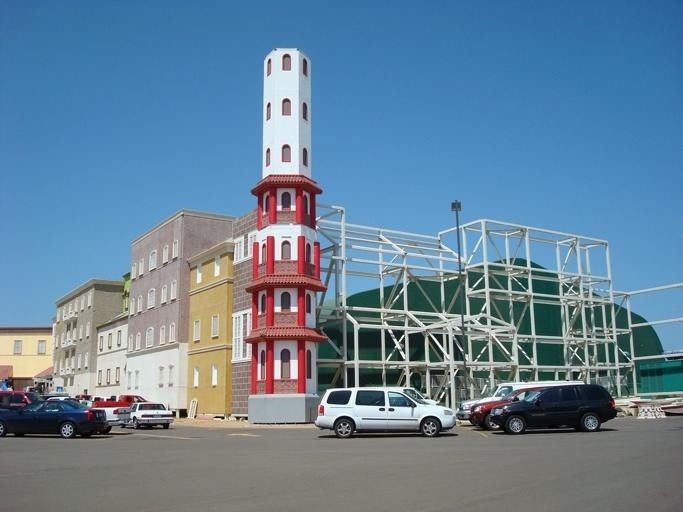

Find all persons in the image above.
[0,377,16,392]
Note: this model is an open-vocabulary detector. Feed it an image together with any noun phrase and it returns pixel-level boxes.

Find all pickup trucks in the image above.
[88,395,160,409]
[24,396,131,435]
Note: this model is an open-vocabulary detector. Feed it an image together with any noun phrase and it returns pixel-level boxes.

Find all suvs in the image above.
[387,387,439,406]
[457,379,586,425]
[490,383,617,434]
[469,386,553,431]
[314,387,455,437]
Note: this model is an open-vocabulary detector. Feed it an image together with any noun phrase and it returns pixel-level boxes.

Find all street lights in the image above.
[449,196,470,399]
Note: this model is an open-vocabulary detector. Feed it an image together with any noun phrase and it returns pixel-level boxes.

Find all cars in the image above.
[120,401,174,430]
[0,380,119,409]
[0,400,107,438]
